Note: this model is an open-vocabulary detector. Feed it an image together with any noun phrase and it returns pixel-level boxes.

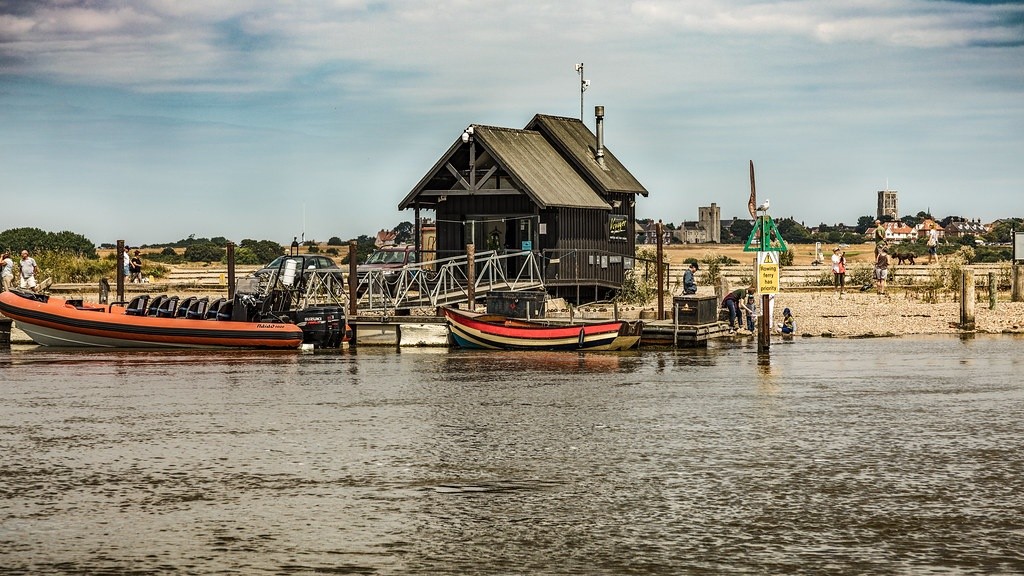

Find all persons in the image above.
[123,245,135,283]
[873,248,889,295]
[722,285,757,334]
[130,250,143,283]
[683,263,700,295]
[18,250,40,291]
[831,248,847,297]
[0,251,14,292]
[781,308,796,340]
[926,222,939,265]
[740,294,757,332]
[874,220,888,261]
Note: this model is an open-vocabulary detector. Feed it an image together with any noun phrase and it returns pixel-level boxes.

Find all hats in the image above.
[124,245,130,250]
[689,262,697,269]
[783,308,791,316]
[833,247,841,253]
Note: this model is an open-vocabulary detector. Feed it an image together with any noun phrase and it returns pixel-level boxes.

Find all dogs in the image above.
[891,252,918,265]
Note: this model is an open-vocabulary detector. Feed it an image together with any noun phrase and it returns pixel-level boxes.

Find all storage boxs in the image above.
[487,291,545,319]
[673,293,717,324]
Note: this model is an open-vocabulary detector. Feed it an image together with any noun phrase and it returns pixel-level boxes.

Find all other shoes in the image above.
[737,327,744,332]
[729,329,734,334]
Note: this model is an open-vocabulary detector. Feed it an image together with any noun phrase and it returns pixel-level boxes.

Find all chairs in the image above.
[124,293,233,322]
[384,252,398,262]
